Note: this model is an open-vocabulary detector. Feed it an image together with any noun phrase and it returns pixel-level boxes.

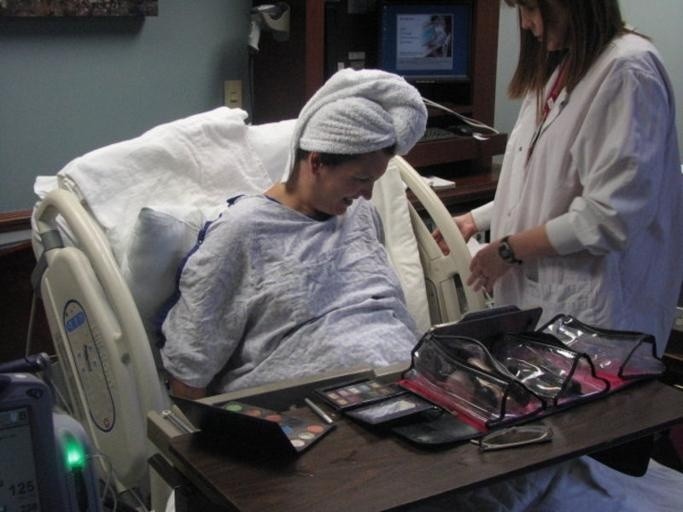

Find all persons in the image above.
[159,66,429,400]
[431,0,683,358]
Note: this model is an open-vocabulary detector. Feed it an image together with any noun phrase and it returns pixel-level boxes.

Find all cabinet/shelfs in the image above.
[253,1,508,169]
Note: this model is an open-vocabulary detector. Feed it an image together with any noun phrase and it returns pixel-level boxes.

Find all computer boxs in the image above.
[325,0,376,83]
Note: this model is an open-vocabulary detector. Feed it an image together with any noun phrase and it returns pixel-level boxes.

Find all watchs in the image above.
[498,235,524,267]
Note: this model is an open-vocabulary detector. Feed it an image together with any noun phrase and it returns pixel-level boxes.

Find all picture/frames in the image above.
[382,4,469,77]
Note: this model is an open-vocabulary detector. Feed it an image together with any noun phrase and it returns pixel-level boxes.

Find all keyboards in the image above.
[418,127,458,143]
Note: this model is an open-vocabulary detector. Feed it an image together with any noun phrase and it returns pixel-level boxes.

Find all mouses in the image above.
[447,125,473,136]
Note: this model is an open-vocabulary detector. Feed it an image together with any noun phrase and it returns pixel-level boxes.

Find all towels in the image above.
[280,68,428,184]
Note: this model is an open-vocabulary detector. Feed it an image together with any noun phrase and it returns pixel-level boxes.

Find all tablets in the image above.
[427,306,543,360]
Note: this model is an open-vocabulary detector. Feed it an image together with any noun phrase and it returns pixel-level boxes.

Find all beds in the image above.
[31,118,682,512]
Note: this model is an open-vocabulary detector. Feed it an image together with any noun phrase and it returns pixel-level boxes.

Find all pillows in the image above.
[121,208,202,384]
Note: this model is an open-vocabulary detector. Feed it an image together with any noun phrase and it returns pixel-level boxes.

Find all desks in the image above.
[167,367,683,512]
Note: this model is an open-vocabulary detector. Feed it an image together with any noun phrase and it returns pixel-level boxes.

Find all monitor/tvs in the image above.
[0,372,64,512]
[376,0,477,111]
